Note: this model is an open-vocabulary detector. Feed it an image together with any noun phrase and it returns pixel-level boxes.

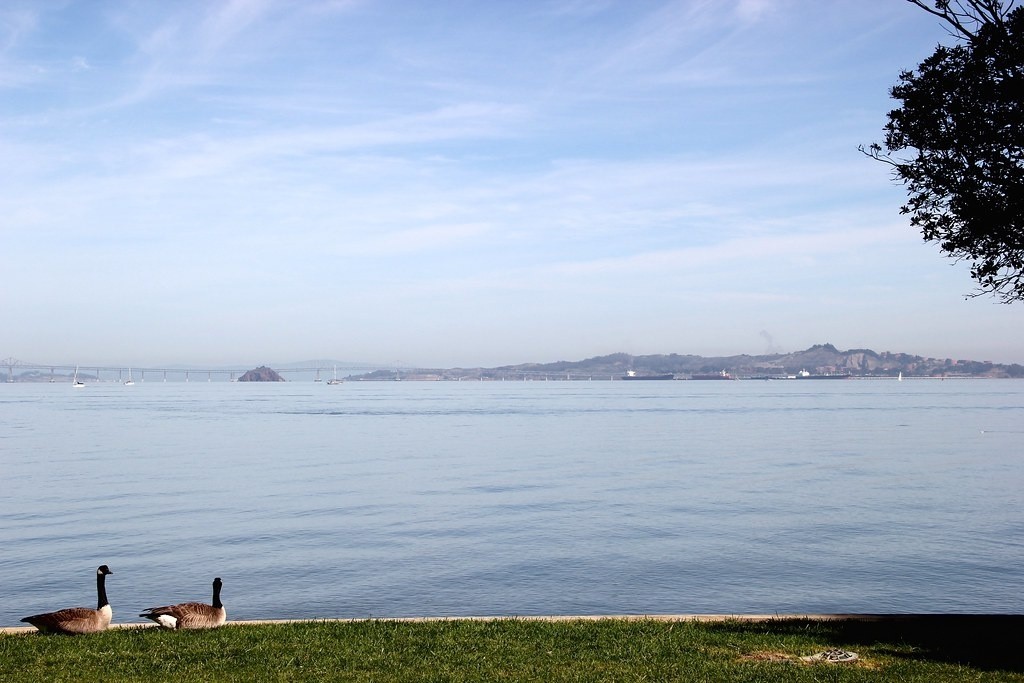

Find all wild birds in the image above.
[19,564,114,633]
[138,577,227,630]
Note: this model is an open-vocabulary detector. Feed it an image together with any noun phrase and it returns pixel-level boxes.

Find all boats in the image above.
[692,368,735,380]
[621,369,674,380]
[796,367,852,379]
